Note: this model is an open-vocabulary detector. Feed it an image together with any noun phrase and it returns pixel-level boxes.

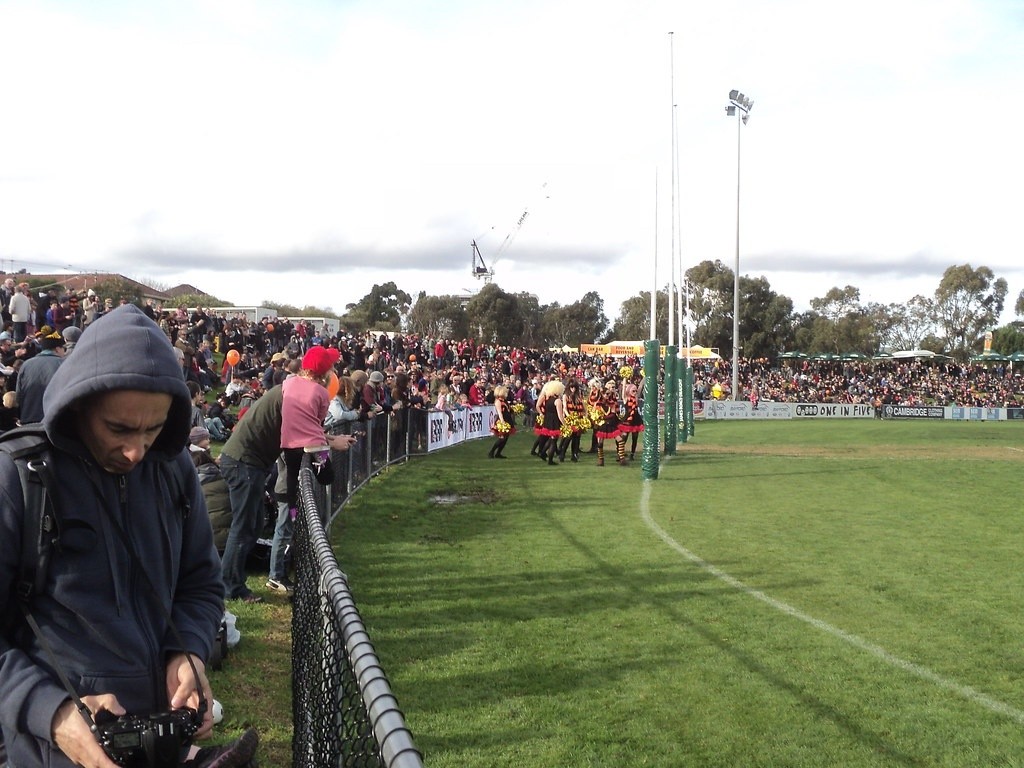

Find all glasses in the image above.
[610,386,616,389]
[385,374,394,379]
[632,389,637,392]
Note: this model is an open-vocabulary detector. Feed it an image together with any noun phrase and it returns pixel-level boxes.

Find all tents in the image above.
[969,351,1024,369]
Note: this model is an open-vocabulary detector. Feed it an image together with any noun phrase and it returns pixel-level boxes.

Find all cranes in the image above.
[469,209,530,287]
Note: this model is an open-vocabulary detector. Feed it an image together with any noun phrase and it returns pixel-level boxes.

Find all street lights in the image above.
[724,89,755,398]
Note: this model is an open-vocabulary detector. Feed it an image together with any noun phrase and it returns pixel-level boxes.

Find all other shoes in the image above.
[596,458,604,466]
[619,458,626,466]
[488,453,494,458]
[185,731,258,768]
[495,454,507,458]
[215,434,226,442]
[531,447,581,465]
[220,427,231,437]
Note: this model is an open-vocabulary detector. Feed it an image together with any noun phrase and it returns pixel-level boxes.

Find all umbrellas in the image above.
[782,350,893,361]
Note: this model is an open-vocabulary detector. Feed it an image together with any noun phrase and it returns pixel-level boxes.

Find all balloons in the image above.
[327,373,340,401]
[227,350,240,367]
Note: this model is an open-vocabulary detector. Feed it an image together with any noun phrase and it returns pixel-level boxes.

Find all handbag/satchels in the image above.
[208,610,240,671]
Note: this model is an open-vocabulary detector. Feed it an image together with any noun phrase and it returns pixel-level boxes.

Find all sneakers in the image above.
[266,575,294,592]
[236,590,262,603]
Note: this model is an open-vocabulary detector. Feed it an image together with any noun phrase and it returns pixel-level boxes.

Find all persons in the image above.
[266,452,294,593]
[218,383,357,603]
[738,358,1024,407]
[0,279,323,455]
[0,303,225,768]
[323,317,733,503]
[281,345,340,520]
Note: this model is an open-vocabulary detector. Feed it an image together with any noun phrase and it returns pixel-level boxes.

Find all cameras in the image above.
[349,431,366,443]
[93,702,204,768]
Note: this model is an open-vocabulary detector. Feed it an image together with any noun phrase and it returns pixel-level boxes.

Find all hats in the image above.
[87,289,95,297]
[370,370,384,382]
[189,426,210,445]
[63,326,82,342]
[351,370,368,384]
[271,352,288,363]
[302,345,340,376]
